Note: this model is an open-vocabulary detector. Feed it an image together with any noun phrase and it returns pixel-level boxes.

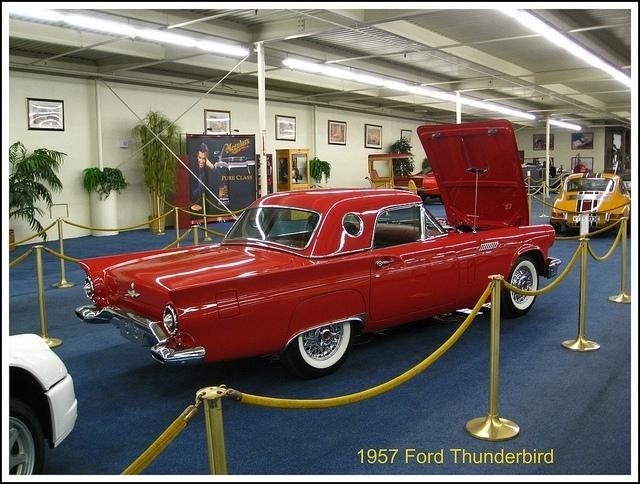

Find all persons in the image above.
[189,142,219,212]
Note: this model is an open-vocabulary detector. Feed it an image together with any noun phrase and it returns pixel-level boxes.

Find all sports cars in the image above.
[70,117,564,379]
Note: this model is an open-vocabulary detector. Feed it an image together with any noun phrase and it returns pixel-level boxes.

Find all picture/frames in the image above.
[400,129,413,149]
[533,133,554,151]
[328,120,347,145]
[26,96,65,132]
[571,132,594,149]
[364,123,383,149]
[274,114,297,142]
[571,156,594,174]
[203,109,231,136]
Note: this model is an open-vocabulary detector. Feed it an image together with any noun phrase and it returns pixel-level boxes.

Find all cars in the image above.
[213,143,255,177]
[394,166,443,205]
[574,136,592,147]
[8,330,79,474]
[551,170,630,237]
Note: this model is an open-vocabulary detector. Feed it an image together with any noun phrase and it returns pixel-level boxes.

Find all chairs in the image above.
[344,220,358,235]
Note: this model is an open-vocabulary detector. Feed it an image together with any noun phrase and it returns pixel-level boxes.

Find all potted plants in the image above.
[131,110,184,234]
[9,141,69,252]
